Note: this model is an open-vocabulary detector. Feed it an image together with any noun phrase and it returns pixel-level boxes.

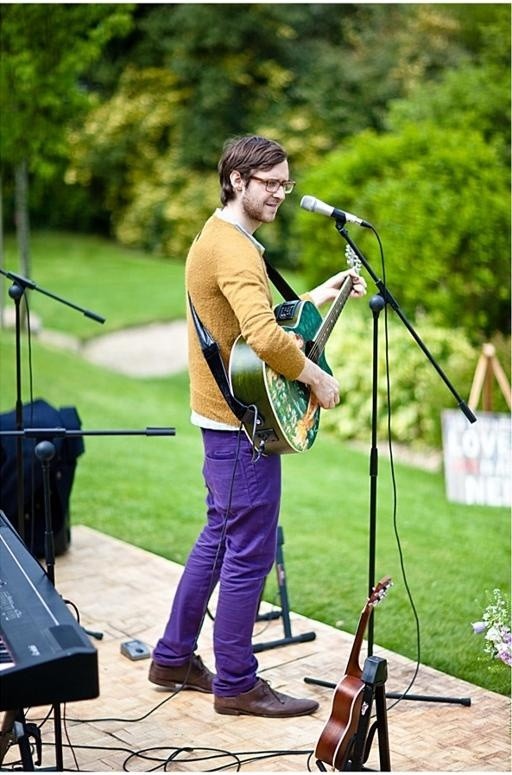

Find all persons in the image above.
[144,133,367,721]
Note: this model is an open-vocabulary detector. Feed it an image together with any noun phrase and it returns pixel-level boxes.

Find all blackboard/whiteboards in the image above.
[441,409,511,507]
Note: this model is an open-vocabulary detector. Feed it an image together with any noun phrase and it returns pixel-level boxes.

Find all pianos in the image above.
[2,510,100,710]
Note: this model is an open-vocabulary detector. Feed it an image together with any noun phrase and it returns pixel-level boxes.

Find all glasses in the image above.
[250,175,296,194]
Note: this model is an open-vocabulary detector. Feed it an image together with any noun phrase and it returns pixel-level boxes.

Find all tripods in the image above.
[301,294,473,771]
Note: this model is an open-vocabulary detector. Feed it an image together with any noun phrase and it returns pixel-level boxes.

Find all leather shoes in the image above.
[148,655,216,694]
[213,679,319,718]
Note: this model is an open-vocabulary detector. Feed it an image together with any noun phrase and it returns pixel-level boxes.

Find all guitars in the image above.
[228,244,362,454]
[315,576,394,771]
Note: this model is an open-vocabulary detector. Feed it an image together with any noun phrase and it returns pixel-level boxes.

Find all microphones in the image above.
[299,193,359,234]
